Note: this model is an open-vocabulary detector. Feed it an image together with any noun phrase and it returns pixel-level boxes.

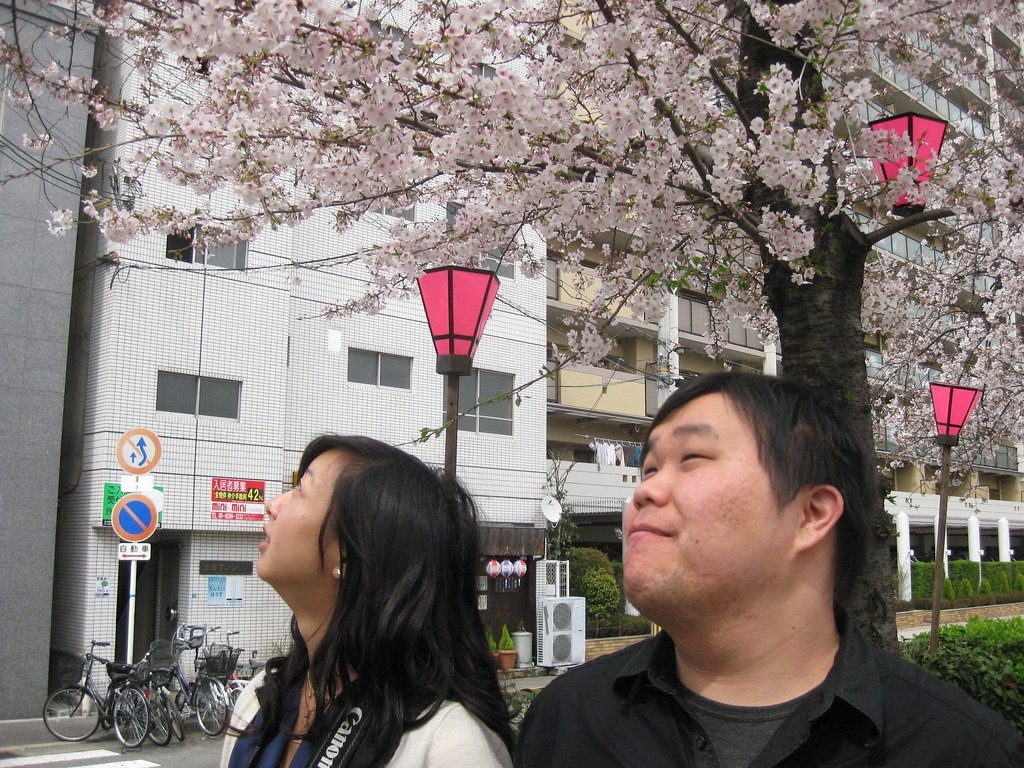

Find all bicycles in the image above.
[40,620,269,757]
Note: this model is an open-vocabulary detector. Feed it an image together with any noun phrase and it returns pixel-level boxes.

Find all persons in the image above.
[218,431,522,768]
[515,367,1024,768]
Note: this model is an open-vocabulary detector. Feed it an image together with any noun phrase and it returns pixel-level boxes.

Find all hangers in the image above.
[594,438,642,448]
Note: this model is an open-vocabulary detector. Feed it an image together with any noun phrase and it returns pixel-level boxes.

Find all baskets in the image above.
[149,639,172,671]
[58,655,86,685]
[209,643,239,675]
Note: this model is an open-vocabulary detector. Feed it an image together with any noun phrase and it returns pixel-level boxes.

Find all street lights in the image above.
[415,266,501,486]
[925,380,985,661]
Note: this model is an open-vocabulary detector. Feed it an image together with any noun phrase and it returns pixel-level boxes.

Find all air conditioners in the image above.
[536,596,587,667]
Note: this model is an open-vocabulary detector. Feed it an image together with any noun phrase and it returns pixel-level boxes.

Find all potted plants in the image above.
[485,624,498,662]
[497,623,518,670]
